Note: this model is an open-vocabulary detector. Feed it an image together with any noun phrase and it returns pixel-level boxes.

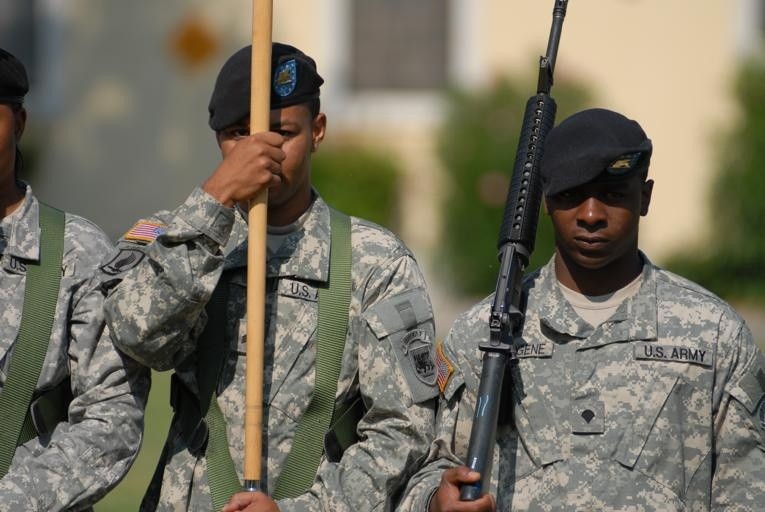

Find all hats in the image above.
[540,107,652,198]
[210,41,325,131]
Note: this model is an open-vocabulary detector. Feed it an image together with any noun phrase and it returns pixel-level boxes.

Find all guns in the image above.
[455,1,569,501]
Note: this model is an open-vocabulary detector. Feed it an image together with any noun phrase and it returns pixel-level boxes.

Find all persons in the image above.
[393,106,765,511]
[0,48,150,512]
[97,43,437,512]
